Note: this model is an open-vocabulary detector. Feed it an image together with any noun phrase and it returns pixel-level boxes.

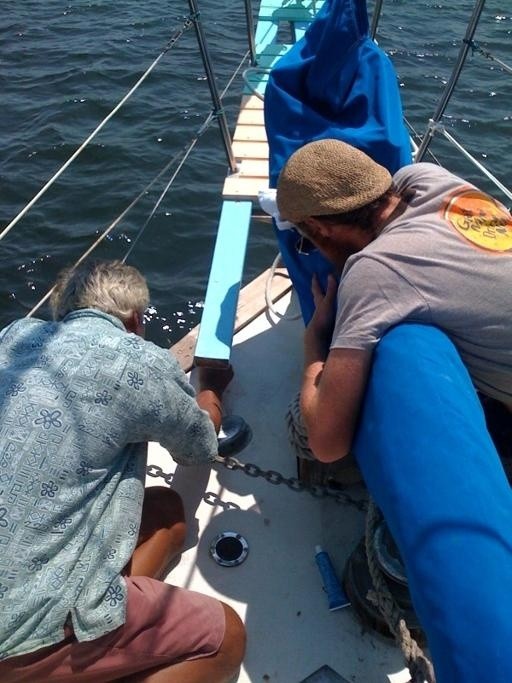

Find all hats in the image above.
[276,139,393,225]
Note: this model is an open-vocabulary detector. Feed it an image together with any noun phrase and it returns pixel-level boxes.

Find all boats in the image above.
[117,264,438,682]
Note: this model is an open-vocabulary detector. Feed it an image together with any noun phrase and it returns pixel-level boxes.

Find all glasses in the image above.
[294,232,320,256]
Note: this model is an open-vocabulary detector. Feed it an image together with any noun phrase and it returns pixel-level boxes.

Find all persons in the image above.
[0,261,248,681]
[277,137,512,485]
